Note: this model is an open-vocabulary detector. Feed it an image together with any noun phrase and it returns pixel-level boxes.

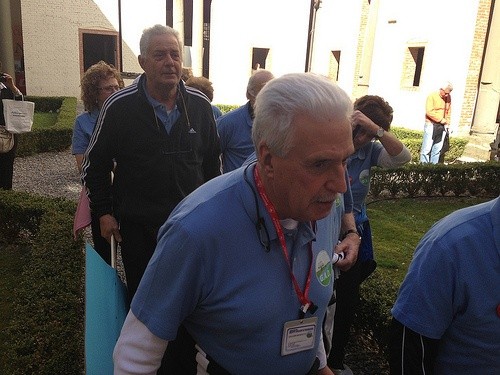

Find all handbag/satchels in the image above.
[2,91,35,133]
[432,123,444,144]
[0,125,14,153]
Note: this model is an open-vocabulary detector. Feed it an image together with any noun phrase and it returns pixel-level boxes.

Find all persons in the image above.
[113,73,355,375]
[80,25,223,375]
[71,60,411,375]
[420,82,454,163]
[0,72,25,191]
[389,195,500,375]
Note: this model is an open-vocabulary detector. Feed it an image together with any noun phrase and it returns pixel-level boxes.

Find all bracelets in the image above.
[342,229,362,240]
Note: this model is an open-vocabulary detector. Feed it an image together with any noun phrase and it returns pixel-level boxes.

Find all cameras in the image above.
[0,76,7,82]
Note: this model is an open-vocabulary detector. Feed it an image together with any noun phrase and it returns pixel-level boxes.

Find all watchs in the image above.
[375,126,384,139]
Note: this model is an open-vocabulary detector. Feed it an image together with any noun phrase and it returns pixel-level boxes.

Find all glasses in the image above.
[159,126,195,156]
[95,85,119,91]
